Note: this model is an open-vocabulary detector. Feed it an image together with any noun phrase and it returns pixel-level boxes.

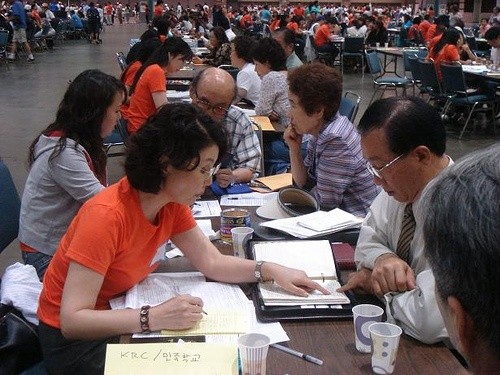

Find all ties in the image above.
[395,202,416,264]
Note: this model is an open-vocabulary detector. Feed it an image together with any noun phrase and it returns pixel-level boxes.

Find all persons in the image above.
[127,37,195,135]
[251,37,314,163]
[284,62,380,221]
[135,0,500,130]
[188,67,262,188]
[422,142,500,375]
[37,101,331,375]
[230,35,261,106]
[0,0,131,62]
[335,95,470,370]
[19,70,128,283]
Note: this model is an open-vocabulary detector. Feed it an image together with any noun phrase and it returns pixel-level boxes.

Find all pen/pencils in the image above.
[227,197,257,200]
[153,278,208,315]
[269,344,324,366]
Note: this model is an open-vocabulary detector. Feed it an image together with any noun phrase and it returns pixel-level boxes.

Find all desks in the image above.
[168,63,215,79]
[372,44,405,87]
[462,64,500,134]
[113,194,469,375]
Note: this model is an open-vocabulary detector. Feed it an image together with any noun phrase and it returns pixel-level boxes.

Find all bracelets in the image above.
[140,305,151,334]
[255,261,265,283]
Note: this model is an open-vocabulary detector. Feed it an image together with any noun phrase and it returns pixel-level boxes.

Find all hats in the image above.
[256,188,318,219]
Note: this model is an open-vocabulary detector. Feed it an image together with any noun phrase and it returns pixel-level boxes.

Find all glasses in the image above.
[365,146,412,179]
[194,90,230,115]
[180,158,221,181]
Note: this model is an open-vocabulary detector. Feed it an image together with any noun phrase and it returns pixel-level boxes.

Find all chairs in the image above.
[0,16,499,375]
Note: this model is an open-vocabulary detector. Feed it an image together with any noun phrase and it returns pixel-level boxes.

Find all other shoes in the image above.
[24,58,35,62]
[3,57,16,62]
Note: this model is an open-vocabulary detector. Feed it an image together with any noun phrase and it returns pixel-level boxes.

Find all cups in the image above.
[237,332,270,375]
[385,43,388,48]
[221,208,251,242]
[351,304,384,353]
[230,227,257,259]
[368,322,403,374]
[376,42,380,48]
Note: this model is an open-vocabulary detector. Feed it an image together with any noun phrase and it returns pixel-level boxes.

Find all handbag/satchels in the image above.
[0,303,44,375]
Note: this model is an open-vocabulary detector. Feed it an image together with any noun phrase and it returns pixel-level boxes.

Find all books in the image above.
[246,218,360,322]
